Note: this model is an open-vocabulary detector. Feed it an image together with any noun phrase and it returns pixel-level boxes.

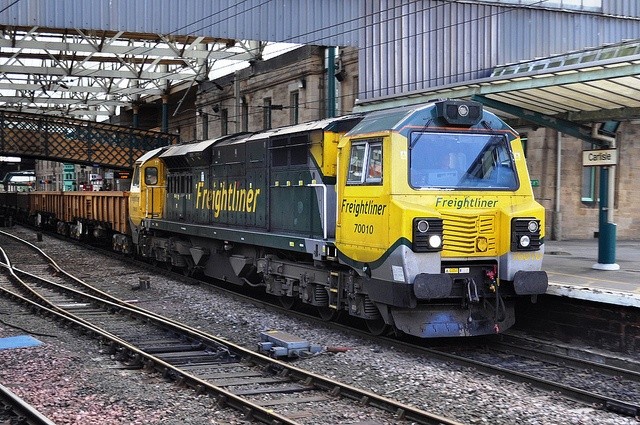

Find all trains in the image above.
[0,97,549,351]
